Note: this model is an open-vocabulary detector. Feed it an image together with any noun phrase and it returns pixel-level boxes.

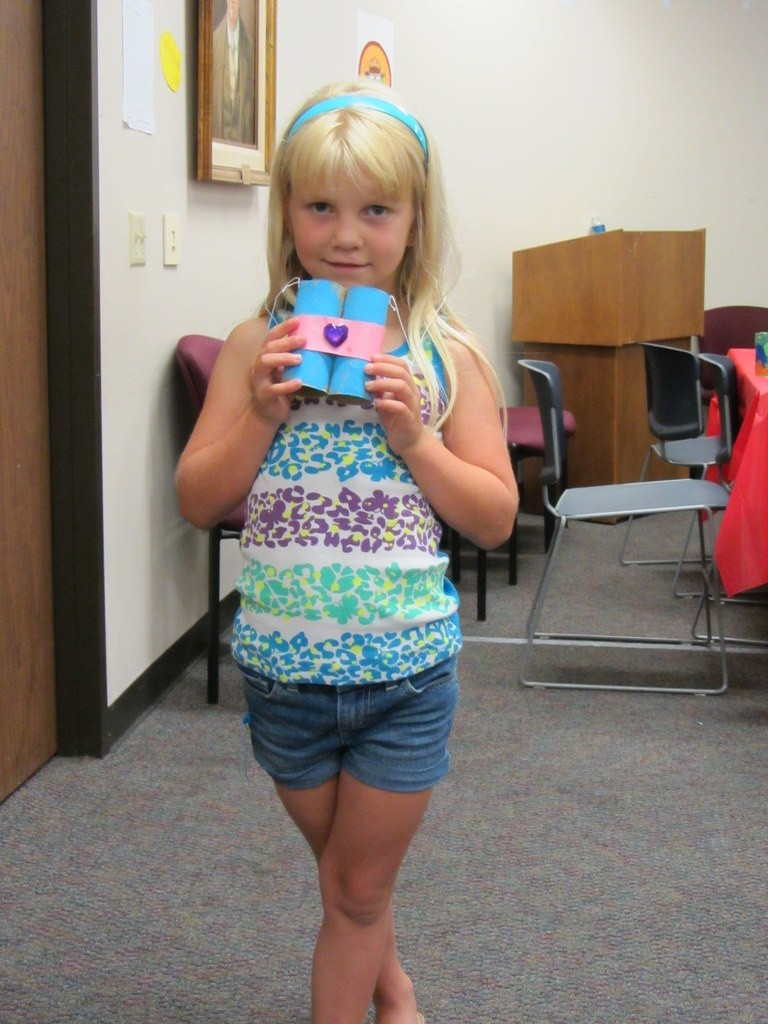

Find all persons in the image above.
[175,80,519,1024]
[213,0,254,144]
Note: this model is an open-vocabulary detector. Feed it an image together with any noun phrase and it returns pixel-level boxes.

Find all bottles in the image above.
[590,215,606,234]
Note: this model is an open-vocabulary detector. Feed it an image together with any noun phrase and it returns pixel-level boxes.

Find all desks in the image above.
[699,348,768,597]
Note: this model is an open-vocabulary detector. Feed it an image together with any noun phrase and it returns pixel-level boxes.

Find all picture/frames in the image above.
[196,0,277,187]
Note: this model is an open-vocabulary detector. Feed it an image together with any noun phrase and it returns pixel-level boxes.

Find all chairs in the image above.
[440,406,577,624]
[176,334,247,708]
[519,307,768,695]
